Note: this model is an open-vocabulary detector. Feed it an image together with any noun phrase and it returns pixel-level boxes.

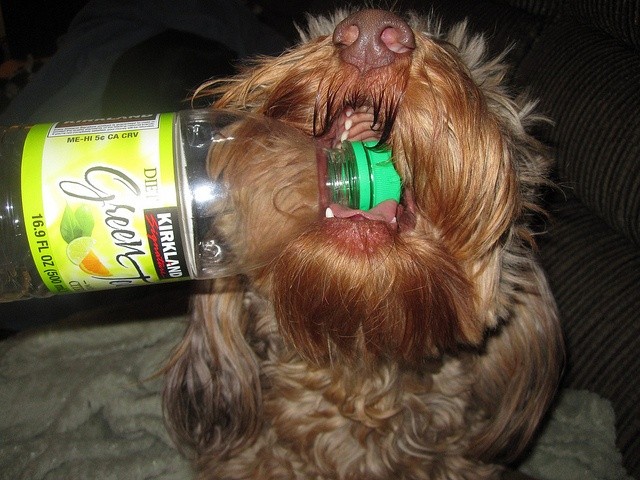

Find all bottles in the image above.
[1,109,402,305]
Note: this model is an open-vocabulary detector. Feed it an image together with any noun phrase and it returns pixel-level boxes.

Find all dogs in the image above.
[146,6,567,480]
